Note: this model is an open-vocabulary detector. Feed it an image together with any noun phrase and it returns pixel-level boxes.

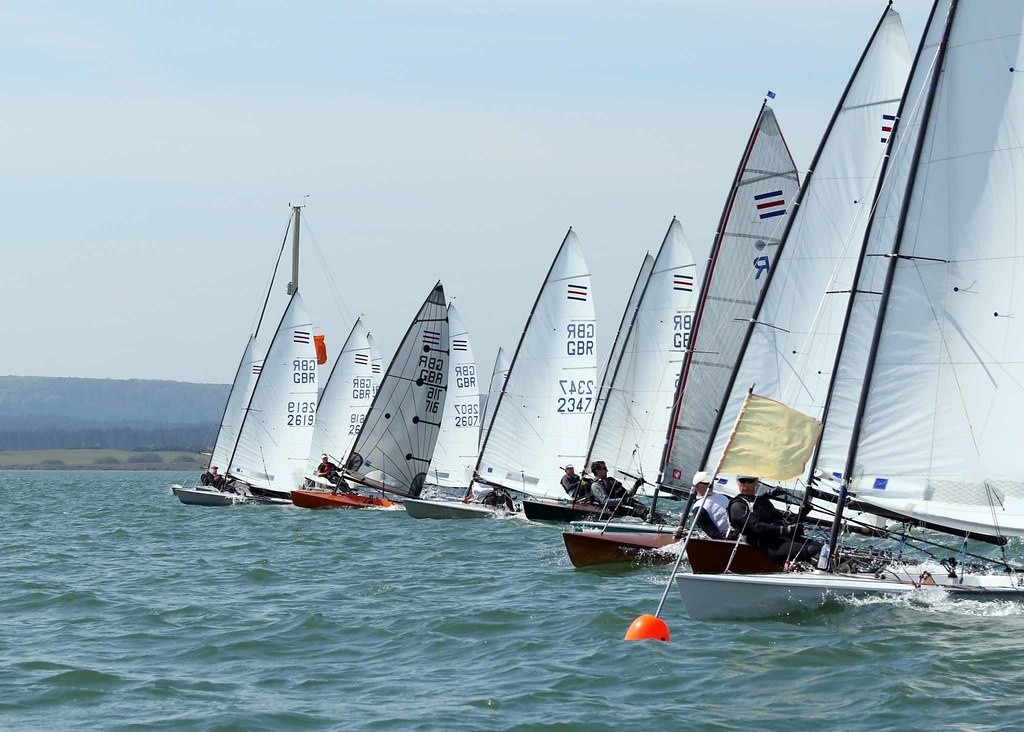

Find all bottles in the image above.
[817,539,830,569]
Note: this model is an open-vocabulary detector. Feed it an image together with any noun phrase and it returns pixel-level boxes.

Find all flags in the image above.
[715,392,822,482]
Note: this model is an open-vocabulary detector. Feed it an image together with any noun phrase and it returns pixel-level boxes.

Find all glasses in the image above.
[737,478,758,484]
[596,467,606,470]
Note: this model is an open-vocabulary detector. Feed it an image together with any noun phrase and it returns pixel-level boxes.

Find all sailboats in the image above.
[173,196,704,535]
[675,0,1024,620]
[560,90,895,570]
[674,1,913,576]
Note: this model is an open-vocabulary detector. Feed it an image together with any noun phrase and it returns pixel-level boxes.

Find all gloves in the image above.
[635,478,646,486]
[770,486,789,497]
[784,523,804,536]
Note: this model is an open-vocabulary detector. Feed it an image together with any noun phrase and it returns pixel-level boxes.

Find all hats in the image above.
[321,455,327,459]
[211,466,218,469]
[693,472,712,486]
[566,464,574,469]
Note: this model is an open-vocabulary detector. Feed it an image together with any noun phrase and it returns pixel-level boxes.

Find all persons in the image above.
[317,453,353,494]
[589,460,668,525]
[207,466,234,492]
[560,463,591,498]
[692,470,740,540]
[727,475,823,554]
[471,480,516,516]
